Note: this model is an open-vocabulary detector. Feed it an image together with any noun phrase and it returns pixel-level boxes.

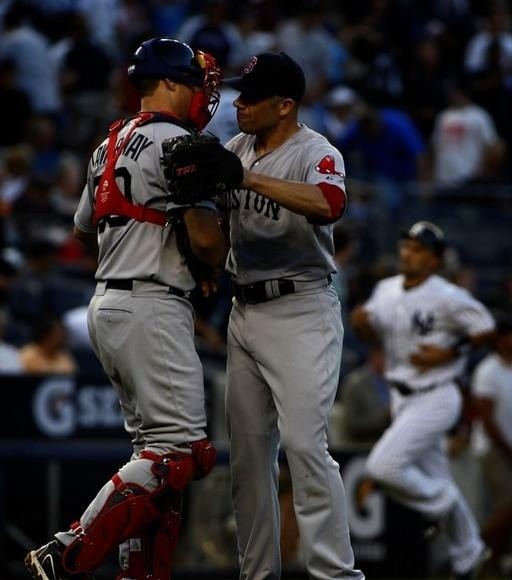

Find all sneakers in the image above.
[456,548,493,580]
[24,538,67,580]
[425,512,453,541]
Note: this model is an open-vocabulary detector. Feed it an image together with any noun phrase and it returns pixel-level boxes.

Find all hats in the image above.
[223,51,305,103]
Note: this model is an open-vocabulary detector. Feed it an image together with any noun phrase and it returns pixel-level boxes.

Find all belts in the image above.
[234,278,292,306]
[105,280,184,298]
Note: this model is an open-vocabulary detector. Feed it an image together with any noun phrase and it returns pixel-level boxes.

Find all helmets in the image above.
[400,220,448,256]
[130,38,221,136]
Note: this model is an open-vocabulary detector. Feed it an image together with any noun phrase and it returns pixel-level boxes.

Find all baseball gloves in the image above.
[162,134,242,205]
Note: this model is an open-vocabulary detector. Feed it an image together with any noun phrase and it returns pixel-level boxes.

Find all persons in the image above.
[0,0,512,375]
[444,318,512,562]
[23,37,227,580]
[164,50,362,580]
[349,219,494,580]
